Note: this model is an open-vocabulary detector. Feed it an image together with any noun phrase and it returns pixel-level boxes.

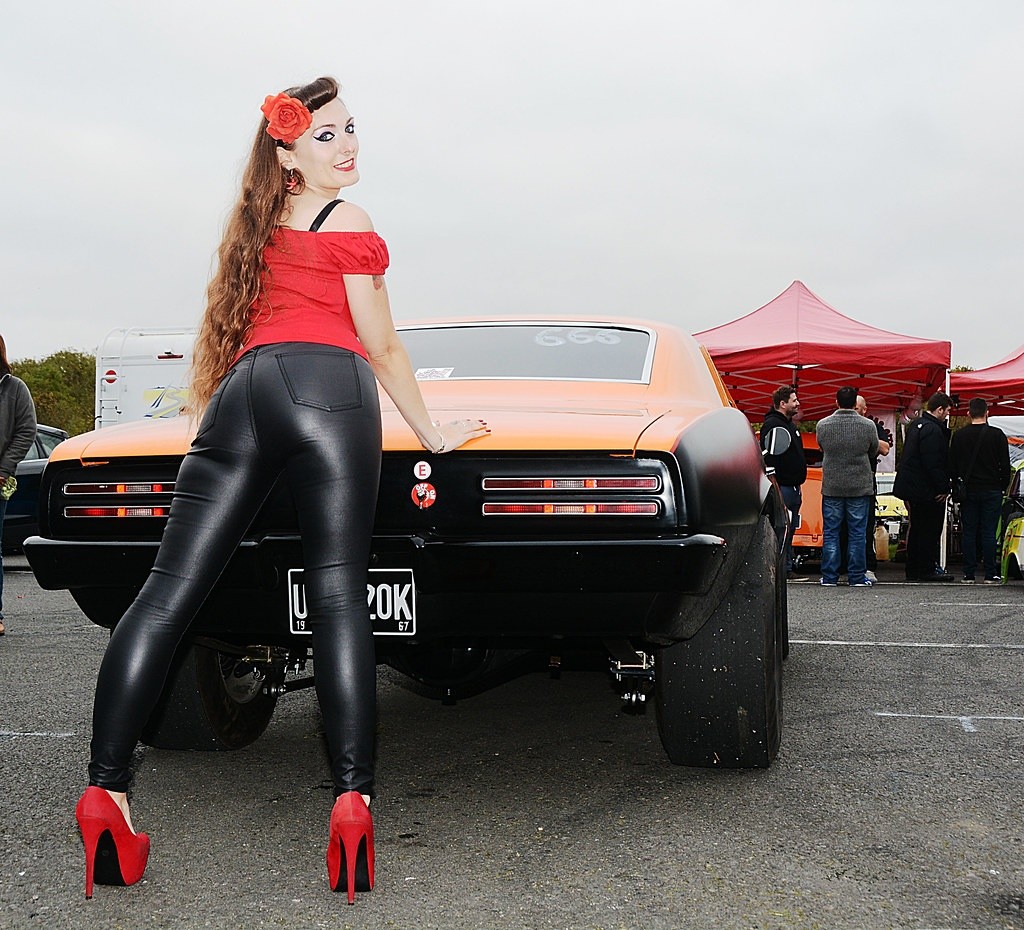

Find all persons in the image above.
[0,337,36,637]
[816,385,890,587]
[893,392,954,582]
[758,384,810,583]
[950,398,1010,583]
[73,74,490,907]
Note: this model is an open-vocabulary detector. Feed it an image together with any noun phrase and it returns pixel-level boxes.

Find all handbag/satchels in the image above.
[950,474,967,502]
[892,422,946,504]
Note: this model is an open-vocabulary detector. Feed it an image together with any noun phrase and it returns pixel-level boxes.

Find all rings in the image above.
[460,421,466,428]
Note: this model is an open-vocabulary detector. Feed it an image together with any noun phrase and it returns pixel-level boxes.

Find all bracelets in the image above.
[431,430,445,454]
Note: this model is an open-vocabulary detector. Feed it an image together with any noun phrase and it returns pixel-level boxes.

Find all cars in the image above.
[2,423,72,542]
[992,459,1024,583]
[875,471,909,522]
[23,309,790,773]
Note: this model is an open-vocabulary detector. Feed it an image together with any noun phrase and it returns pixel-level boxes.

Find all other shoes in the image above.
[905,575,919,583]
[984,571,1002,583]
[960,575,976,584]
[864,569,878,583]
[850,578,873,587]
[819,577,837,587]
[922,572,954,582]
[786,571,809,582]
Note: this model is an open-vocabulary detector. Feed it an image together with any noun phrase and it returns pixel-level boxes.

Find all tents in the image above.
[940,345,1024,572]
[692,279,953,431]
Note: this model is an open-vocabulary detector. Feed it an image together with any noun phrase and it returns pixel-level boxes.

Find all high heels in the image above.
[326,791,374,906]
[75,786,150,900]
